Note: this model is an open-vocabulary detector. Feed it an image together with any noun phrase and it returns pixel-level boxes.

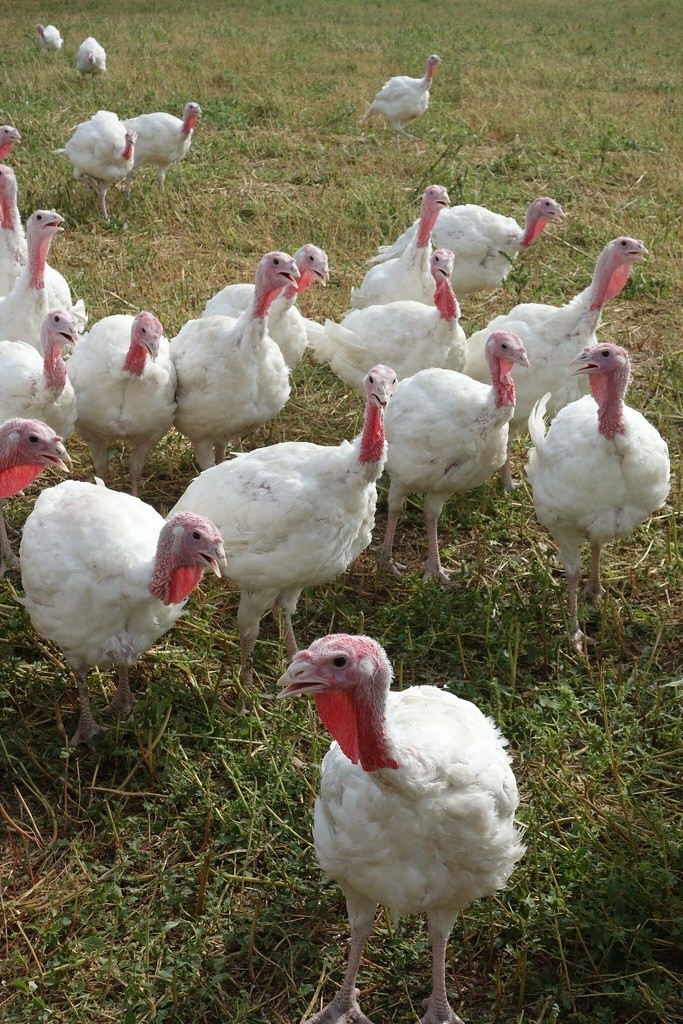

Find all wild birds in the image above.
[0,124,682,758]
[49,100,203,225]
[36,24,64,60]
[276,631,530,1024]
[77,36,107,82]
[358,52,442,146]
